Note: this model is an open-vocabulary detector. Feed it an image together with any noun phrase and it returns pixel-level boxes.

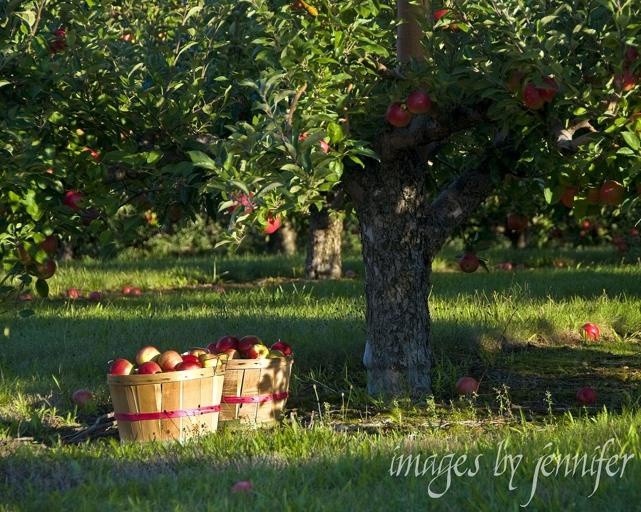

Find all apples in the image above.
[576,385,596,404]
[73,390,92,408]
[107,335,294,375]
[232,480,250,492]
[18,10,640,301]
[454,376,478,396]
[580,323,600,341]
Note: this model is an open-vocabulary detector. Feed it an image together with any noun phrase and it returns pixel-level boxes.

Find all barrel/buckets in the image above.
[220,353,293,427]
[105,366,225,445]
